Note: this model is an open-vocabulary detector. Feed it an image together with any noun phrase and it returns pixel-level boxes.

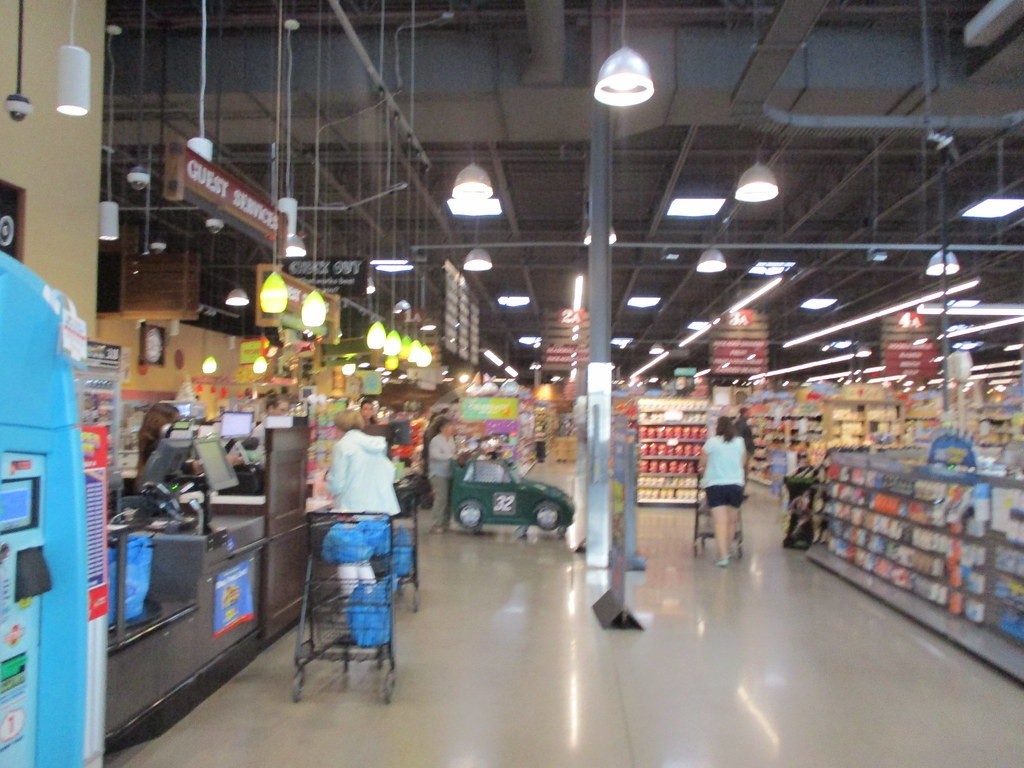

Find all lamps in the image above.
[56,1,1024,368]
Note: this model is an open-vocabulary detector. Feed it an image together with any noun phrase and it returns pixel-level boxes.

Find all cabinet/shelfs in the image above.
[512,436,552,478]
[637,399,1024,505]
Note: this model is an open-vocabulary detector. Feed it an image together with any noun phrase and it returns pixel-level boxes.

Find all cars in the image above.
[452,459,576,539]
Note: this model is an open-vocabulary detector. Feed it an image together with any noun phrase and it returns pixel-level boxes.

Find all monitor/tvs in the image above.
[221,411,253,437]
[139,436,239,489]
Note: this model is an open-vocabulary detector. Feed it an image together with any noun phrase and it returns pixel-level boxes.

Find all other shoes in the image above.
[716,555,733,568]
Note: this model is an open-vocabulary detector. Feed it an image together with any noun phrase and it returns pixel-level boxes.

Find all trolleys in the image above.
[290,511,401,704]
[693,473,744,561]
[388,486,421,612]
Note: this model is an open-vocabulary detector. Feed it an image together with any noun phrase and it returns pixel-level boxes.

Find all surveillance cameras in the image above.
[205,219,224,233]
[150,242,167,253]
[126,173,150,190]
[3,95,33,122]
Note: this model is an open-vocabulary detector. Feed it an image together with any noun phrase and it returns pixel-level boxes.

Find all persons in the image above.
[553,418,577,463]
[420,408,455,509]
[733,408,753,489]
[535,423,547,464]
[358,400,377,426]
[428,415,469,534]
[326,410,400,580]
[246,394,291,496]
[697,416,748,566]
[137,402,244,495]
